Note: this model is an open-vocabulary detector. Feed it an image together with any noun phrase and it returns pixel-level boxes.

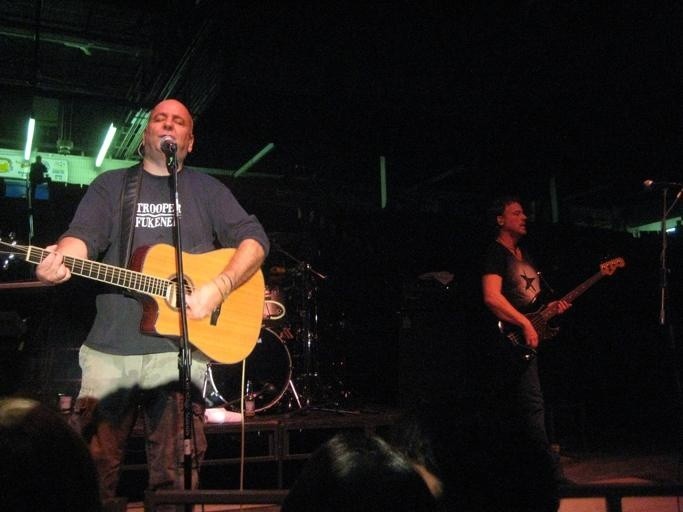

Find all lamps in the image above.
[24,113,37,163]
[94,120,118,171]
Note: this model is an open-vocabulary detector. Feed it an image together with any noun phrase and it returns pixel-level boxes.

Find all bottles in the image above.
[244,380,256,417]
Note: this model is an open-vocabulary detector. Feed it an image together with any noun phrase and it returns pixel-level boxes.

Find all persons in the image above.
[0,397,101,512]
[35,98,271,512]
[479,197,579,494]
[27,155,52,199]
[279,428,456,511]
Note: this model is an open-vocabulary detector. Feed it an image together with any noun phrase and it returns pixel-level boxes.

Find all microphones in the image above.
[641,180,683,190]
[159,135,178,153]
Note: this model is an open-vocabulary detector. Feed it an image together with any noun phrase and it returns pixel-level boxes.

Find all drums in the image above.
[208,325,293,413]
[263,286,285,321]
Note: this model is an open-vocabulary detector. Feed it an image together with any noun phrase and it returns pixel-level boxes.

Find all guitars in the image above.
[0,233,265,365]
[497,258,626,365]
